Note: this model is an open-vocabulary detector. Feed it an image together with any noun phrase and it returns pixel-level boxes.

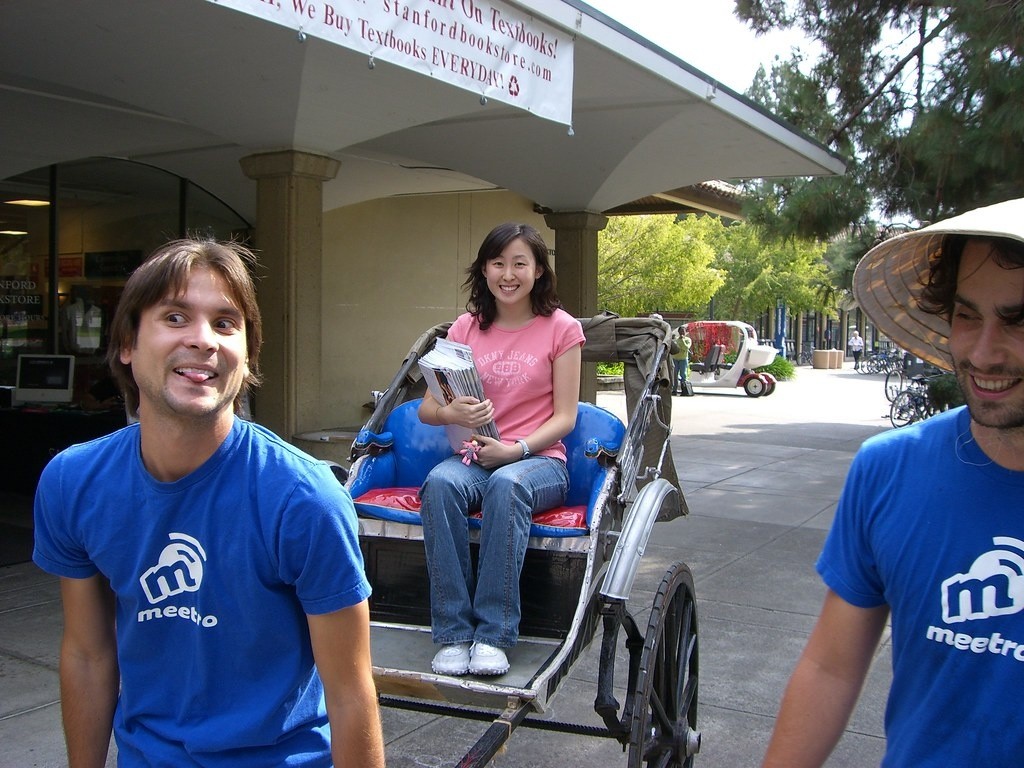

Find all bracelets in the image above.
[436,407,449,425]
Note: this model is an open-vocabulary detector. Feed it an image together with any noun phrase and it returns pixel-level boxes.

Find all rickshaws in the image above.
[342,314,708,767]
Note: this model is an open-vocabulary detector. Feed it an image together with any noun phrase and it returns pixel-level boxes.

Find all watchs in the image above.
[515,440,530,460]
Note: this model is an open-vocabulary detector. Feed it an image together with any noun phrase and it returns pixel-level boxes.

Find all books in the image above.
[418,337,501,454]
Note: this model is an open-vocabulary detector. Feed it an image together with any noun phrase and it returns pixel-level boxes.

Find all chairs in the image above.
[690,344,720,372]
[713,345,726,372]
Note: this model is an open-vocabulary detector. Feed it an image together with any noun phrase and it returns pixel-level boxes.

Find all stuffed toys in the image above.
[460,440,482,466]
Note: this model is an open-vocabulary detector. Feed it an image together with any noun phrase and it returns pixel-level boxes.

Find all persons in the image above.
[672,327,691,396]
[757,197,1024,768]
[418,224,585,675]
[848,331,863,369]
[650,314,679,380]
[34,243,385,768]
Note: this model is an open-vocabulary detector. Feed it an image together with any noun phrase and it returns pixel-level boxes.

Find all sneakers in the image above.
[433,644,470,675]
[469,642,508,675]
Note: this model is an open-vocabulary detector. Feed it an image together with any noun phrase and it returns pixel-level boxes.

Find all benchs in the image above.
[343,397,627,538]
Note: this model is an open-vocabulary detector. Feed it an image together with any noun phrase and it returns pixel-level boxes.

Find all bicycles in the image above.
[854,349,950,429]
[796,346,817,367]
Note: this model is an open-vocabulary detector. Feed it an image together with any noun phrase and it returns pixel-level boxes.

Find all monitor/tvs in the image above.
[15,353,75,402]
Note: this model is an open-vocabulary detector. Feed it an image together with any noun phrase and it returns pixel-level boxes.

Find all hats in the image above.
[852,199,1024,371]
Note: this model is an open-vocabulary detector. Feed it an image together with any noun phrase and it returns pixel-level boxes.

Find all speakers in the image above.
[0,385,19,411]
[75,363,105,400]
